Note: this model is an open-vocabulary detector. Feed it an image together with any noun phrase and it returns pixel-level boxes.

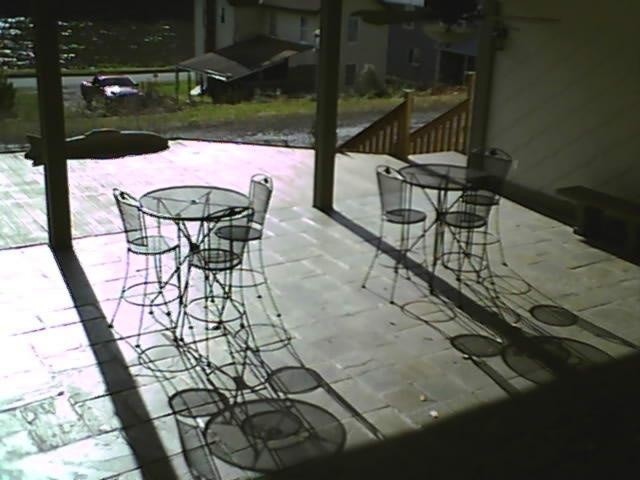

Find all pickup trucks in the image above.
[80,71,148,113]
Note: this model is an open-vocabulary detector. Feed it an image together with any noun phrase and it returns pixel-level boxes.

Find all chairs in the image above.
[361,146,513,310]
[108,173,282,368]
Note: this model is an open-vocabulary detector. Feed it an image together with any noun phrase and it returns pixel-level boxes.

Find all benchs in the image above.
[557,186,640,251]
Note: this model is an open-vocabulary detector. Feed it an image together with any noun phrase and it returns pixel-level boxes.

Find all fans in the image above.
[351,0,562,26]
